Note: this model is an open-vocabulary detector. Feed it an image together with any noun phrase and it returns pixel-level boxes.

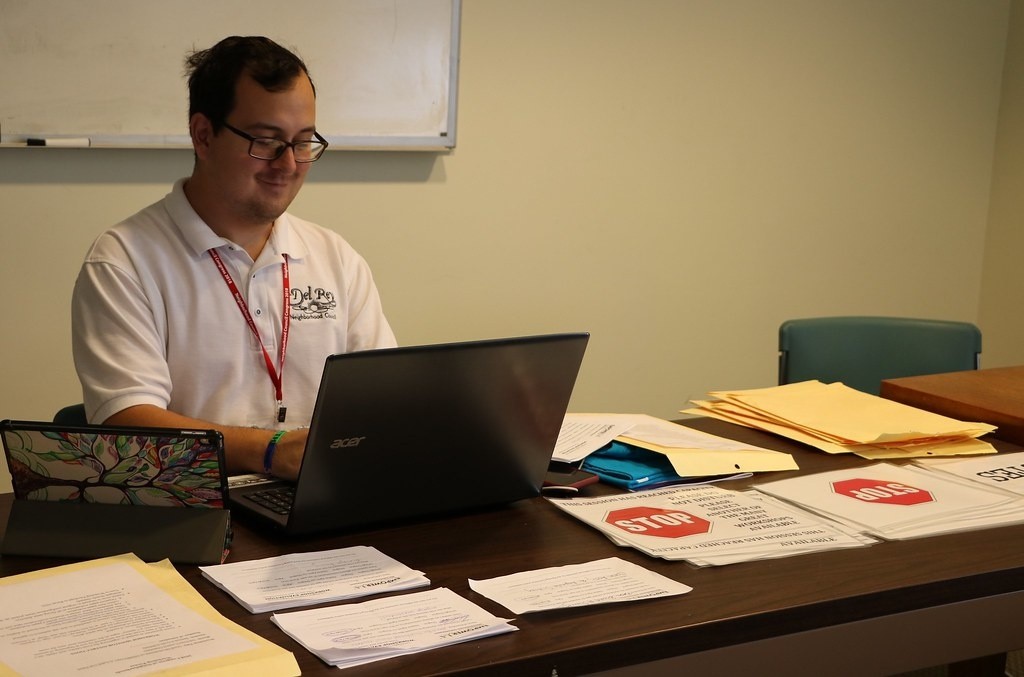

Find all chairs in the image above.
[778,316,982,397]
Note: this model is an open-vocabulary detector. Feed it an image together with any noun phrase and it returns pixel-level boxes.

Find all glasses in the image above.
[217,121,329,163]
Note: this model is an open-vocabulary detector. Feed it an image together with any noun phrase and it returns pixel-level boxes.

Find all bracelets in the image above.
[264,430,286,479]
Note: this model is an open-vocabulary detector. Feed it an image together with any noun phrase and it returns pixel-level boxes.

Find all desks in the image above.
[879,366,1024,447]
[0,416,1024,676]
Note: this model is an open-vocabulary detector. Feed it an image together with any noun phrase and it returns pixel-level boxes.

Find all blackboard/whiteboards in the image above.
[0,0,462,152]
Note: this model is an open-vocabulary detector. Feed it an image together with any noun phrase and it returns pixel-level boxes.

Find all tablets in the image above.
[0,419,232,549]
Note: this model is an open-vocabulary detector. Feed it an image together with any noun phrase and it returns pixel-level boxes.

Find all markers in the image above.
[26,137,91,149]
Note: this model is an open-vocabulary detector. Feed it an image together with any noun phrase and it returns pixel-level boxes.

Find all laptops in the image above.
[220,332,591,546]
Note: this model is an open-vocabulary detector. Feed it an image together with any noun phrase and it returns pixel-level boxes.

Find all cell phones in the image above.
[543,466,599,491]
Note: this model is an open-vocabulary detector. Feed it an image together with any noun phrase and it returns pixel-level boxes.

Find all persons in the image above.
[71,36,398,480]
